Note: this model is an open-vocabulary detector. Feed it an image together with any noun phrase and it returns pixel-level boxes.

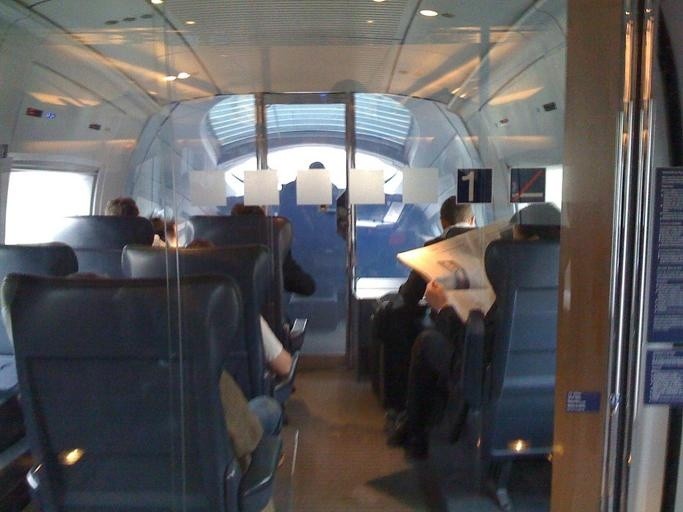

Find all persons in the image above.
[63,271,284,511]
[184,239,294,377]
[229,203,319,296]
[376,201,564,457]
[102,196,182,248]
[374,195,475,410]
[307,161,338,207]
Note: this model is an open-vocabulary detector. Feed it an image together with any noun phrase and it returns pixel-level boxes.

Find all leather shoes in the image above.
[386,429,426,457]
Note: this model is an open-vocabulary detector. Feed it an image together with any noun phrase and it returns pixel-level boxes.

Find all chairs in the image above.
[445,240,564,511]
[120,244,303,434]
[45,214,156,278]
[182,213,307,396]
[2,271,287,512]
[2,240,78,467]
[151,217,172,245]
